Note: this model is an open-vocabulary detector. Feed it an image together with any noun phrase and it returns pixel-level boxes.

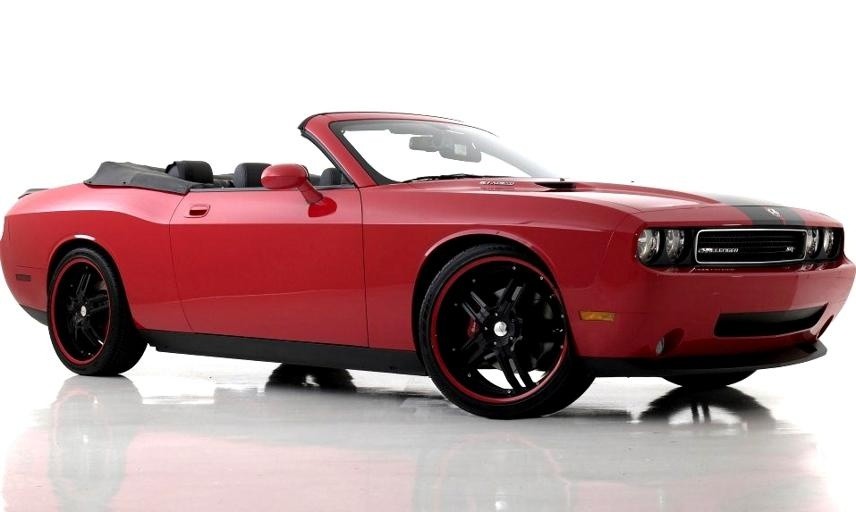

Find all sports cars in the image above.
[0,111,856,420]
[3,366,834,511]
